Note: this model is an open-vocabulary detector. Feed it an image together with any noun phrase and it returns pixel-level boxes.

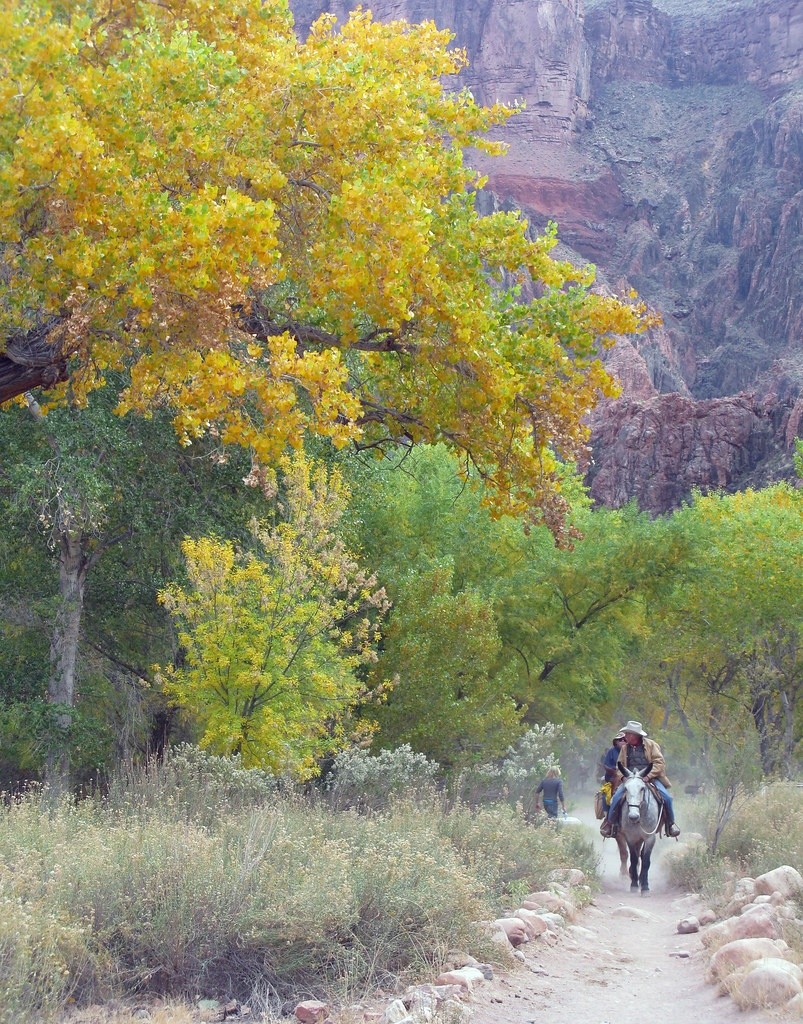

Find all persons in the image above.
[603,731,627,795]
[601,748,610,776]
[535,765,567,826]
[600,719,680,838]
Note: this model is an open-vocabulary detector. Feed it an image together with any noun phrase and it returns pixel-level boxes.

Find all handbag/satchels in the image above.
[593,791,605,819]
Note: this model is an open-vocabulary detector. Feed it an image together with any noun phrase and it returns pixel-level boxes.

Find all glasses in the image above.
[616,738,626,742]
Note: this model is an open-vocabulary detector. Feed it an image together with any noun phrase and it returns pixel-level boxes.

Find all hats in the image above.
[619,721,647,737]
[613,732,626,746]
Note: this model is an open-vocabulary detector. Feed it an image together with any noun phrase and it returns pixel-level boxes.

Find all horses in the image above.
[569,760,666,898]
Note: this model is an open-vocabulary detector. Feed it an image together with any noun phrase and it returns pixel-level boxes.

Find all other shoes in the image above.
[601,823,610,835]
[670,824,680,835]
